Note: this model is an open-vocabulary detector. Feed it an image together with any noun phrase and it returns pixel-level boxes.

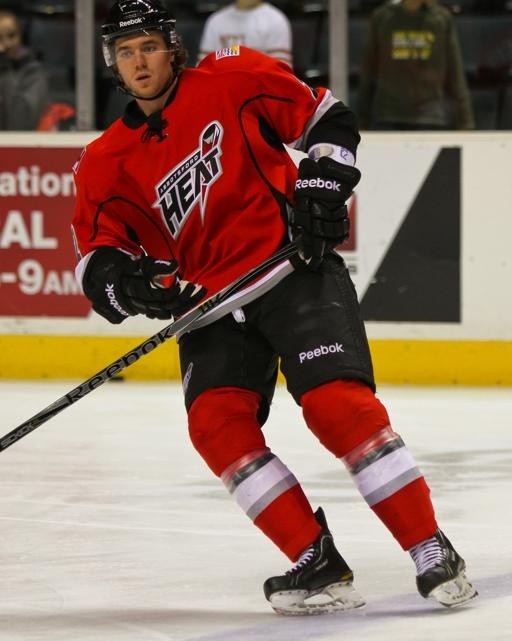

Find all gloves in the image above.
[83,247,207,324]
[294,156,361,264]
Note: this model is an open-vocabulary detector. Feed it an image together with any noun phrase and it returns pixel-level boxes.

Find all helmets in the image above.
[101,0,176,39]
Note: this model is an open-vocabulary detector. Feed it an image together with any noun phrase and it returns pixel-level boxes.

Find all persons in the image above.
[70,1,478,617]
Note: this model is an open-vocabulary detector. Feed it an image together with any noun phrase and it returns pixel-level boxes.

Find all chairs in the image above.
[23,15,511,129]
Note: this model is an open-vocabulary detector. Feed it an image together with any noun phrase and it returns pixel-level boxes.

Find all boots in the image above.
[263,507,354,601]
[410,530,466,598]
